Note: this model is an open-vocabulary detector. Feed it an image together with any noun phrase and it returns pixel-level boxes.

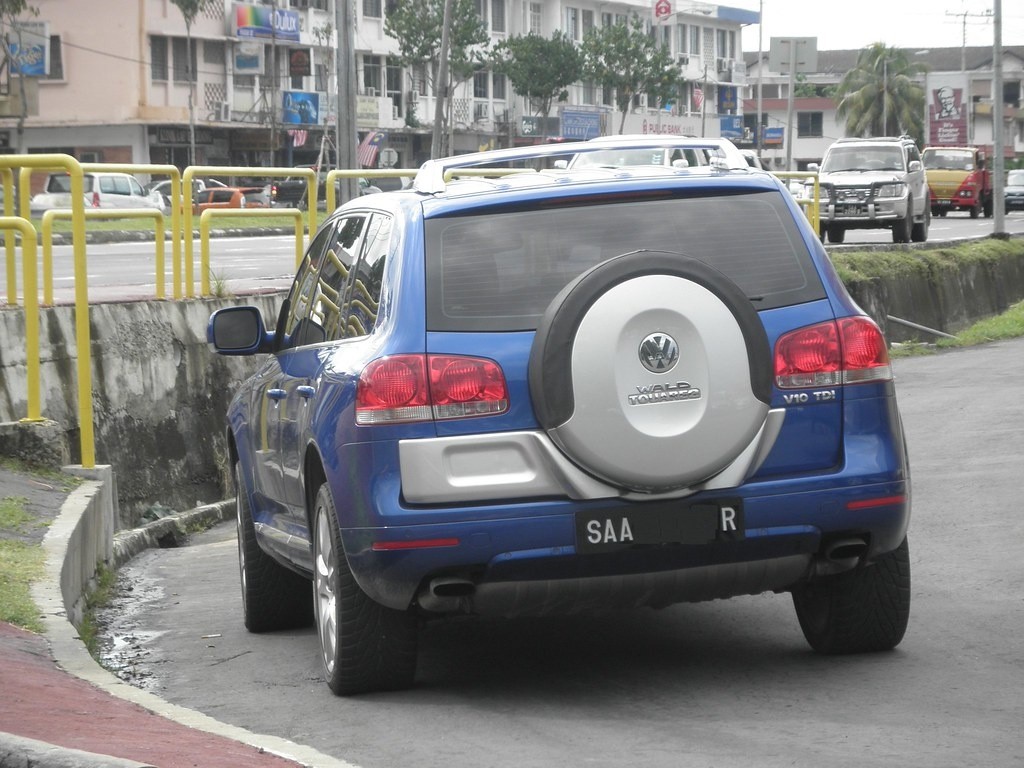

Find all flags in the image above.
[357,130,390,166]
[693,82,704,111]
[286,129,308,147]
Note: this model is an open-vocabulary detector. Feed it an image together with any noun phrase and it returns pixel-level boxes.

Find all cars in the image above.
[32,171,147,209]
[740,150,762,170]
[178,187,269,216]
[141,179,228,207]
[1003,169,1024,210]
[778,176,812,212]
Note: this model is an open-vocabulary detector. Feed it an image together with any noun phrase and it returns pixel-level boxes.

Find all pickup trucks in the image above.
[240,163,340,207]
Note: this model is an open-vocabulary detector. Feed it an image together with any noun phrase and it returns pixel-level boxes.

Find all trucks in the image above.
[920,146,1009,218]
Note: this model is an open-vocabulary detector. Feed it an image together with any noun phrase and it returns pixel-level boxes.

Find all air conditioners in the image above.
[478,102,487,115]
[363,86,376,95]
[408,88,419,101]
[214,100,232,122]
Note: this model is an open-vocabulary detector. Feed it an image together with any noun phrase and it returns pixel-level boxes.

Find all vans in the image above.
[554,135,749,173]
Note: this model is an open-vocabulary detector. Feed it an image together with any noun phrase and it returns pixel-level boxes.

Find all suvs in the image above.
[816,135,930,242]
[208,133,910,693]
